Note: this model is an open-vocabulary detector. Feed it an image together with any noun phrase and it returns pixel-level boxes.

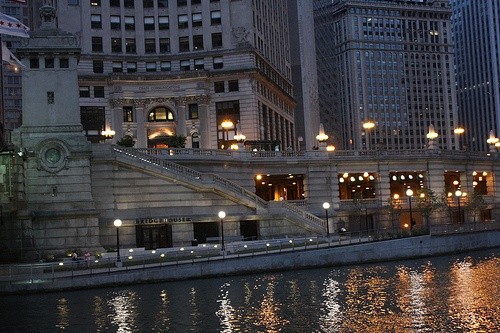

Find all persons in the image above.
[32,249,41,264]
[84,250,91,269]
[72,250,80,265]
[337,217,345,235]
[413,218,417,229]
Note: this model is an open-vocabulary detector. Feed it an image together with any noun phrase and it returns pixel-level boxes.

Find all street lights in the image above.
[454,190,463,225]
[363,121,376,151]
[218,210,227,256]
[323,201,332,243]
[453,128,465,151]
[405,189,415,237]
[113,219,123,267]
[221,120,235,149]
